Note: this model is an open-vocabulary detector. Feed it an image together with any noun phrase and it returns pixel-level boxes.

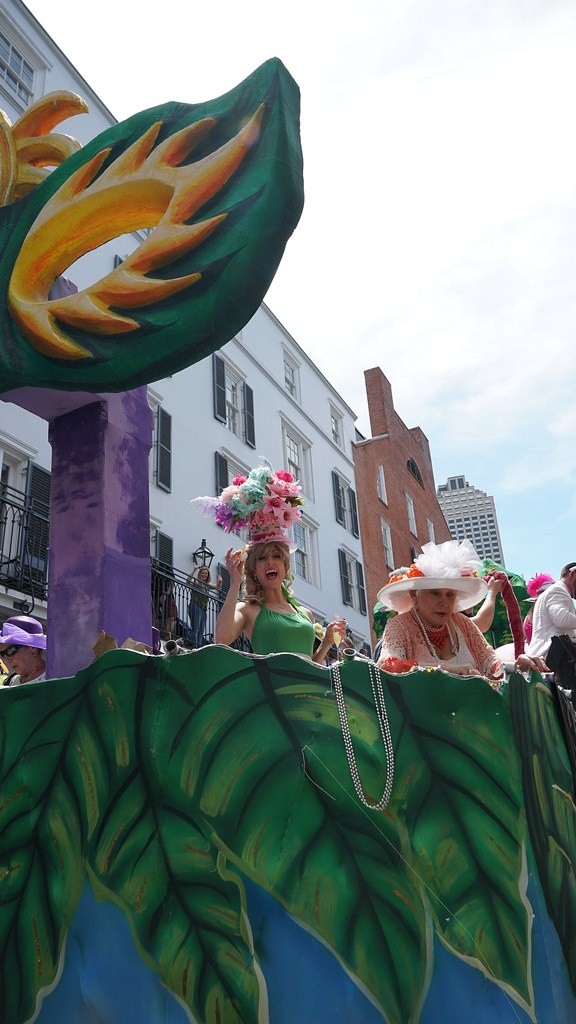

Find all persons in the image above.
[467,575,506,634]
[1,634,50,686]
[185,562,223,650]
[214,539,347,665]
[150,557,178,637]
[376,574,551,680]
[528,561,576,660]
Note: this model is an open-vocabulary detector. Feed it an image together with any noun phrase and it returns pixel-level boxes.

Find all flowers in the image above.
[193,456,309,540]
[385,539,483,586]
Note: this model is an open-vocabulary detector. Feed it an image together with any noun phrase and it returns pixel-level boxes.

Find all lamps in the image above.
[192,539,215,569]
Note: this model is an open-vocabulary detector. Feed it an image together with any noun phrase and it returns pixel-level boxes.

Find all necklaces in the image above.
[265,599,288,609]
[24,659,44,679]
[412,604,461,666]
[411,609,447,648]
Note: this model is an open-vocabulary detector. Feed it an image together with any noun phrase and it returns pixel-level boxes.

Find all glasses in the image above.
[0,644,24,658]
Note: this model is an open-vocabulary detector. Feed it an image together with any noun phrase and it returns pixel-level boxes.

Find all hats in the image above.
[377,539,489,613]
[0,616,47,649]
